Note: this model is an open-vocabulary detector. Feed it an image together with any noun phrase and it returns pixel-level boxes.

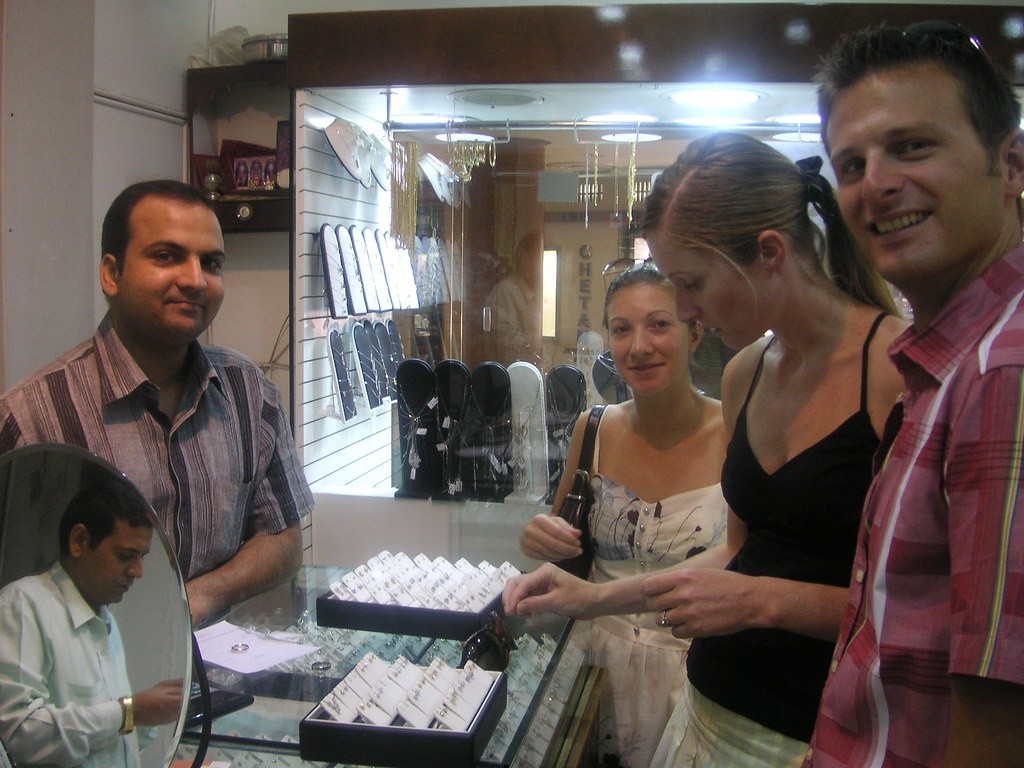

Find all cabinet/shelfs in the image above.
[184,58,293,235]
[169,565,608,768]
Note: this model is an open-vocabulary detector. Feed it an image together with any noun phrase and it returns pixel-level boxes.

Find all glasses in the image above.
[457,612,519,671]
[901,19,1016,128]
[602,257,660,293]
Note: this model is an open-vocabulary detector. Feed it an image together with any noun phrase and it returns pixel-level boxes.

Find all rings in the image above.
[661,610,670,625]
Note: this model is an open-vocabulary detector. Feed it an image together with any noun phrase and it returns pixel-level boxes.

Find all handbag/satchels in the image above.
[557,405,604,576]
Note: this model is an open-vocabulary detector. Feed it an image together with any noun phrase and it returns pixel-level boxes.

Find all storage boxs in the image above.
[315,568,530,641]
[299,670,507,767]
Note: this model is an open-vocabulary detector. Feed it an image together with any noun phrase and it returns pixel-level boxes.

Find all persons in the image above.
[486,232,541,369]
[816,18,1023,767]
[501,133,914,768]
[1,181,316,629]
[0,482,183,768]
[519,268,727,768]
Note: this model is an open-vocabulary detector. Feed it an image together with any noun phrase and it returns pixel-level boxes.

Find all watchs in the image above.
[118,696,133,735]
